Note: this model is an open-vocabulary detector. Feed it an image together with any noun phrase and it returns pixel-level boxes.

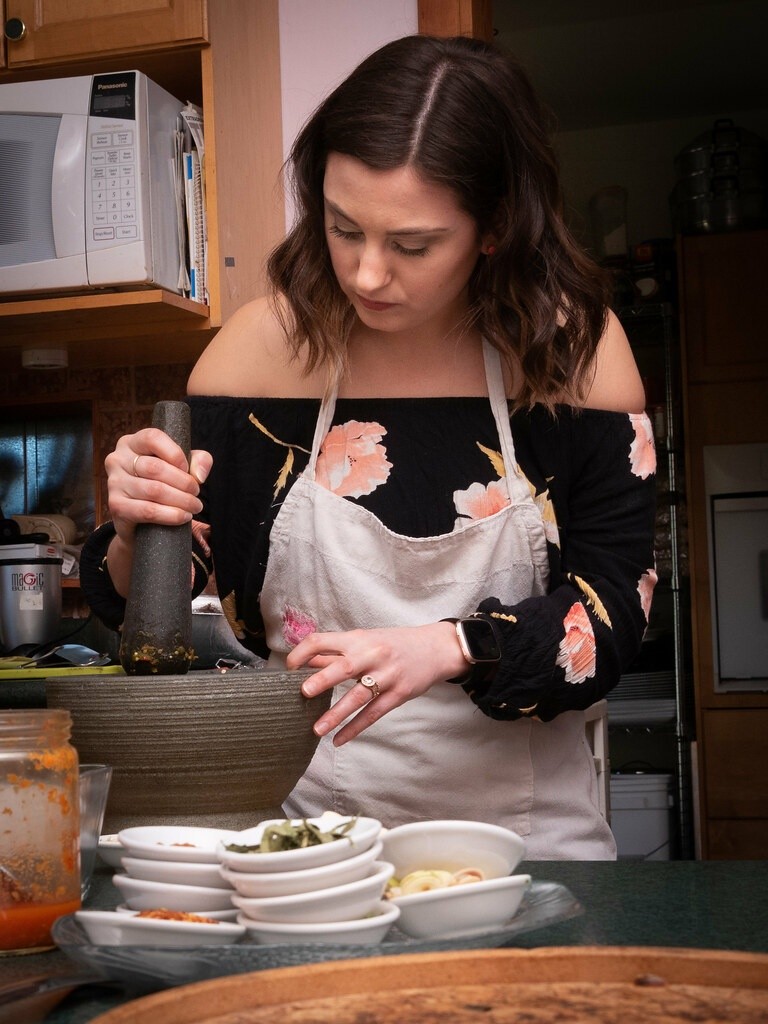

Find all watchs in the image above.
[438,618,502,666]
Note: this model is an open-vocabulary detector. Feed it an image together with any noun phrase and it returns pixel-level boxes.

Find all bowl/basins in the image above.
[75,812,531,949]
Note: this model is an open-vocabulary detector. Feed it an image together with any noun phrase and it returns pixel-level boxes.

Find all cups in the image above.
[0,558,64,657]
[79,762,113,901]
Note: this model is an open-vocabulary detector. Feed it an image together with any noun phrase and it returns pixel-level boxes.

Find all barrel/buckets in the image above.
[609,772,672,861]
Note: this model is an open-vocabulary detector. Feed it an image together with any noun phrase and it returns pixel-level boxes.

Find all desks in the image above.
[44,861,767,1023]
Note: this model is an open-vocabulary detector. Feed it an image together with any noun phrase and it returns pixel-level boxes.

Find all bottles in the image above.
[0,709,83,957]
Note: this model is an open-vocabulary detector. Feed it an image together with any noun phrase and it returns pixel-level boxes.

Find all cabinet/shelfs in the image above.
[678,226,767,863]
[0,0,493,377]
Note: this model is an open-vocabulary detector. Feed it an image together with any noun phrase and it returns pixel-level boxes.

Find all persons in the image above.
[76,34,658,860]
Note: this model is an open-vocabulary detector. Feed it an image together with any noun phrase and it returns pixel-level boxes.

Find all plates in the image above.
[51,883,586,987]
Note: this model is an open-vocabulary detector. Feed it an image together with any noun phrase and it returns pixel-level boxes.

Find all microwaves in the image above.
[0,70,187,295]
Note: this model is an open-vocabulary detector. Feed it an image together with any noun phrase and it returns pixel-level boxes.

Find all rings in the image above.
[133,454,142,477]
[356,674,379,698]
